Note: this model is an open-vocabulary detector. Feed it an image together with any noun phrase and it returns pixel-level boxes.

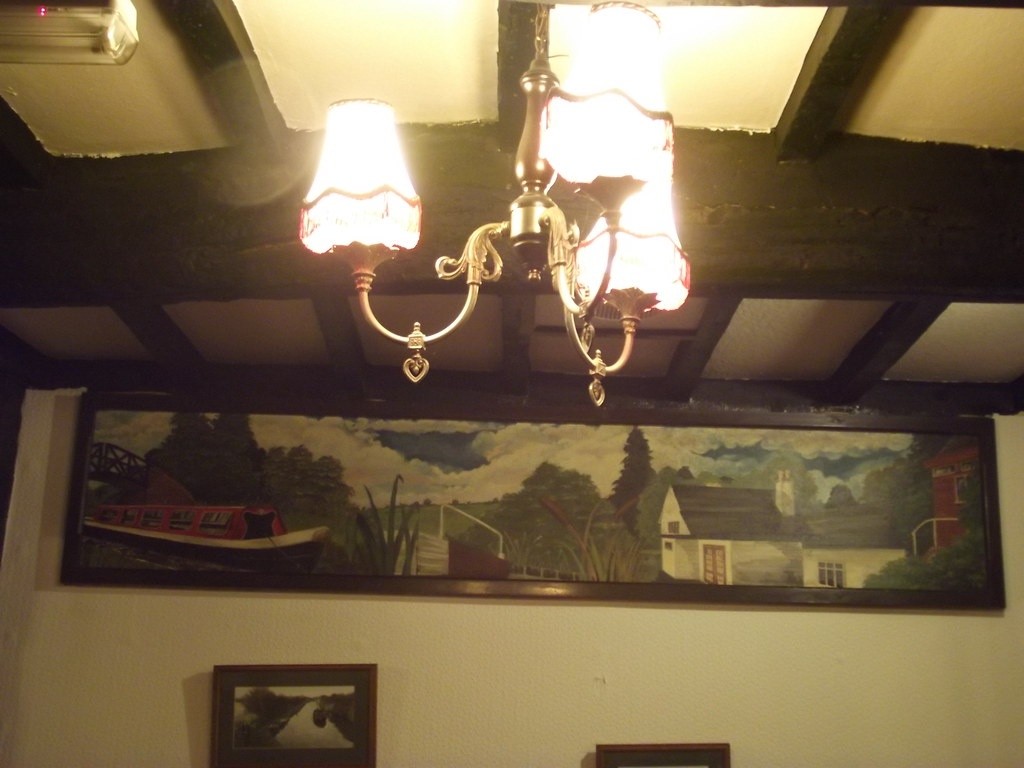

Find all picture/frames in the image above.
[61,378,1007,615]
[596,742,730,768]
[211,664,377,768]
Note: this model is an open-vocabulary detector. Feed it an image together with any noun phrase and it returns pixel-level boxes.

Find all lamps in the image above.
[301,0,689,411]
[0,0,140,65]
